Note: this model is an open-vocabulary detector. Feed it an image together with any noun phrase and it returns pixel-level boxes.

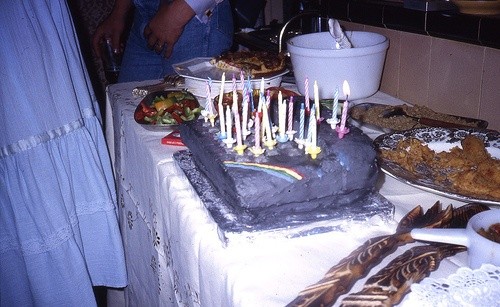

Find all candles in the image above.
[204,68,350,160]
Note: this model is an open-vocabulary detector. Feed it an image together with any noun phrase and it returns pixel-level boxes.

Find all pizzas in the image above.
[210,50,285,75]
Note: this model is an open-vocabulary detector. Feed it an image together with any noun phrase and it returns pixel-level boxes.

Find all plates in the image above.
[374,128,500,205]
[134,90,201,130]
[348,103,489,132]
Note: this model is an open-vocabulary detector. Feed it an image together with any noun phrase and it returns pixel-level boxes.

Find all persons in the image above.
[1,0,128,307]
[92,0,235,83]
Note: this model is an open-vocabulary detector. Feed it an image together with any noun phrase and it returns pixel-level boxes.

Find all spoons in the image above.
[379,106,470,127]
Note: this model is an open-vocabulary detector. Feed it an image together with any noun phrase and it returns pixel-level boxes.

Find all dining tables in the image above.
[105,78,500,307]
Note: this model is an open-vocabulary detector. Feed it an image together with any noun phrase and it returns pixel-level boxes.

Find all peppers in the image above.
[140,99,197,123]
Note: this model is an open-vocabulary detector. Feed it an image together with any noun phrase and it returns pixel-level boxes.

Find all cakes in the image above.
[180,94,379,225]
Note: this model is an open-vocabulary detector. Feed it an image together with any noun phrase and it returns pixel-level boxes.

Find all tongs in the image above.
[131,72,185,96]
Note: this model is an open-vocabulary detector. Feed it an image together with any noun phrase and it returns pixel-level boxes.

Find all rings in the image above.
[158,42,165,48]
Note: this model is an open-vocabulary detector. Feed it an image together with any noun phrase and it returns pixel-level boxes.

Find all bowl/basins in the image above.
[412,209,500,270]
[172,57,290,98]
[287,30,389,101]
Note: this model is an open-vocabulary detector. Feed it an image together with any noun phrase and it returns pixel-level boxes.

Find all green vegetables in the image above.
[144,93,200,124]
